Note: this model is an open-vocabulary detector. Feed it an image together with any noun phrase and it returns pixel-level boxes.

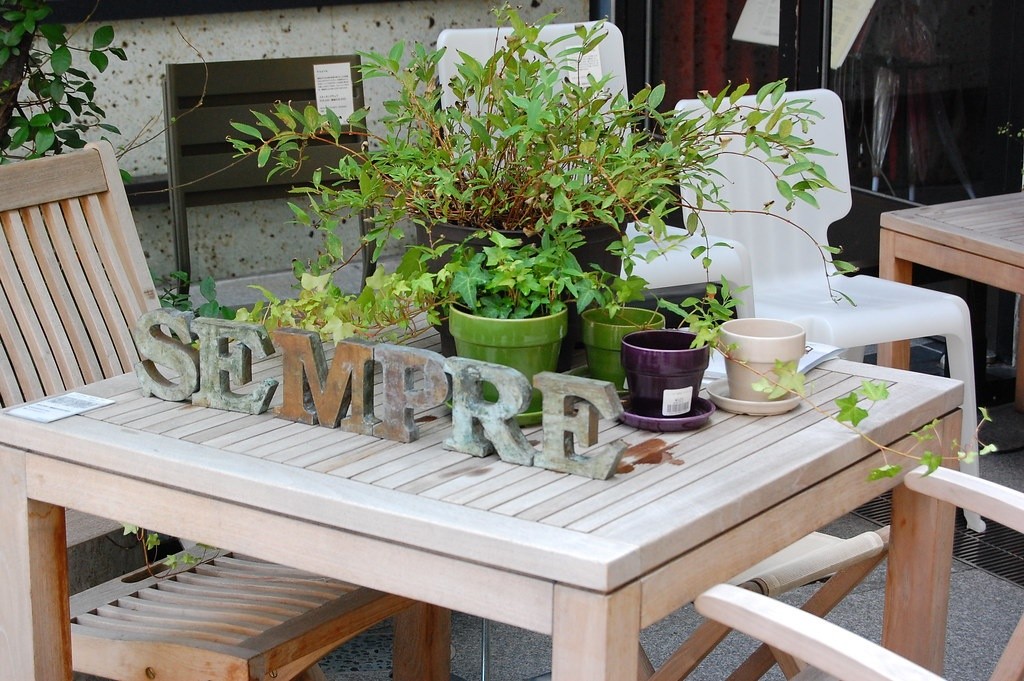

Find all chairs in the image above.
[1,139,450,681]
[437,22,754,319]
[673,88,986,534]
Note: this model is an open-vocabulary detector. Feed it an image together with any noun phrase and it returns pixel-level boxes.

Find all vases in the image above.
[720,318,805,400]
[581,308,666,390]
[620,330,710,419]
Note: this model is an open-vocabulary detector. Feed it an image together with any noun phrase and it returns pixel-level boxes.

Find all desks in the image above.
[0,305,965,681]
[880,192,1024,417]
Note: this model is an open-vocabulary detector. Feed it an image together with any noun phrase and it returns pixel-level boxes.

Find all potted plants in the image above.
[225,2,1000,481]
